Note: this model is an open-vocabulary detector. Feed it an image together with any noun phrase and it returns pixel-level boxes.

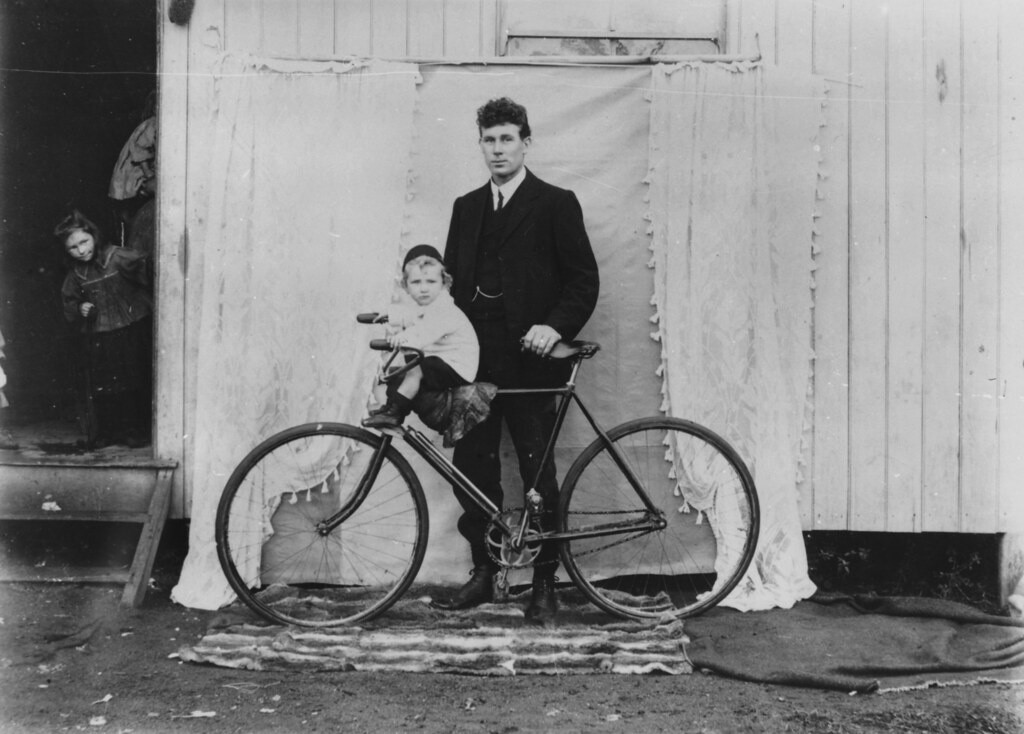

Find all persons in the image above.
[56,215,154,448]
[360,244,479,429]
[428,97,600,619]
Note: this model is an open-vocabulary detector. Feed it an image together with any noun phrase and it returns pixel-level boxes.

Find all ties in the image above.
[497,190,504,211]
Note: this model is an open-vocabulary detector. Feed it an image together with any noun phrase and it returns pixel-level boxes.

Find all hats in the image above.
[402,244,446,268]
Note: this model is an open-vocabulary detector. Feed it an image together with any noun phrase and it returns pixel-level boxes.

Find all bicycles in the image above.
[215,313,760,632]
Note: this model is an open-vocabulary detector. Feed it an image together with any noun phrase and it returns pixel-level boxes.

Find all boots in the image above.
[428,564,493,612]
[523,569,561,624]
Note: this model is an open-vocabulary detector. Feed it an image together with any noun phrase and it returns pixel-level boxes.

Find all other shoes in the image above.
[362,409,400,429]
[95,432,113,448]
[127,429,145,448]
